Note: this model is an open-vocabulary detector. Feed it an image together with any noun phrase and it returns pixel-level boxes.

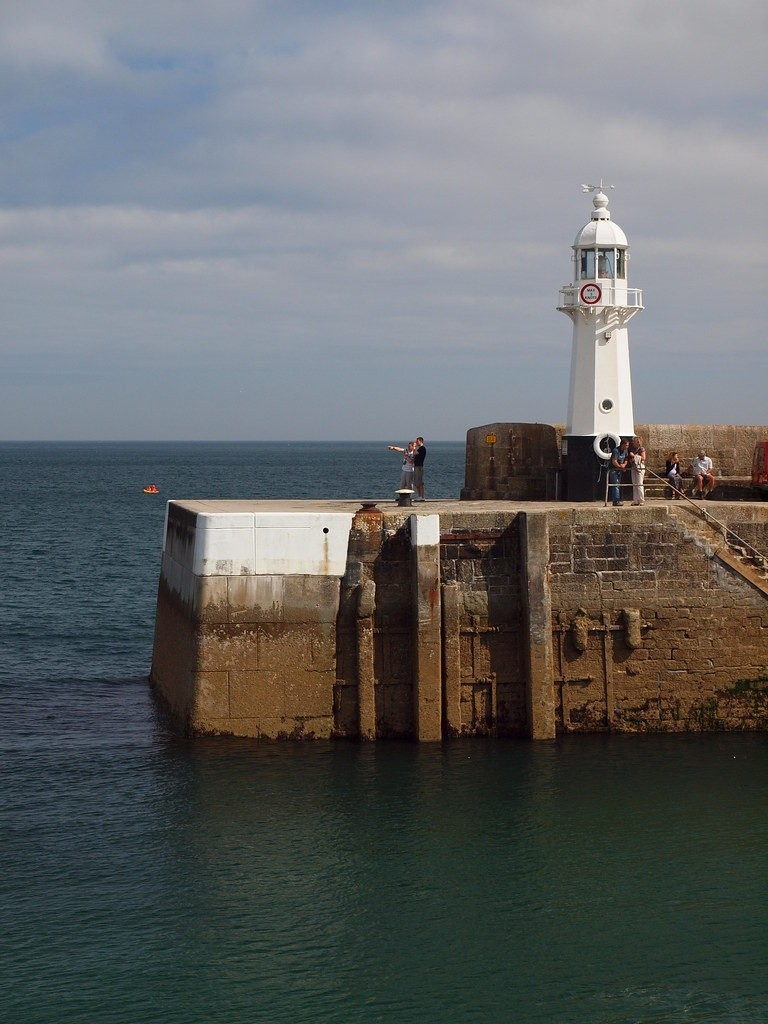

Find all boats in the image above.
[143,488,160,494]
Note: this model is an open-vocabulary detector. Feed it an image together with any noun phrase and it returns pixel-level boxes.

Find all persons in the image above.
[609,438,629,506]
[388,441,416,500]
[413,437,426,500]
[687,450,715,500]
[666,452,685,499]
[146,484,157,492]
[629,436,646,505]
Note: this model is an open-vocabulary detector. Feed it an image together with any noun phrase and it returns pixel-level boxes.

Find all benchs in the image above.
[644,475,754,500]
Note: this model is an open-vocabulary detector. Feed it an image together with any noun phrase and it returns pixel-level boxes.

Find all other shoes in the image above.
[671,492,686,500]
[613,501,624,506]
[631,501,644,506]
[698,490,713,499]
[415,496,425,502]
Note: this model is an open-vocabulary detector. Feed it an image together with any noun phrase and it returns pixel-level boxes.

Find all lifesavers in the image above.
[593,432,622,460]
